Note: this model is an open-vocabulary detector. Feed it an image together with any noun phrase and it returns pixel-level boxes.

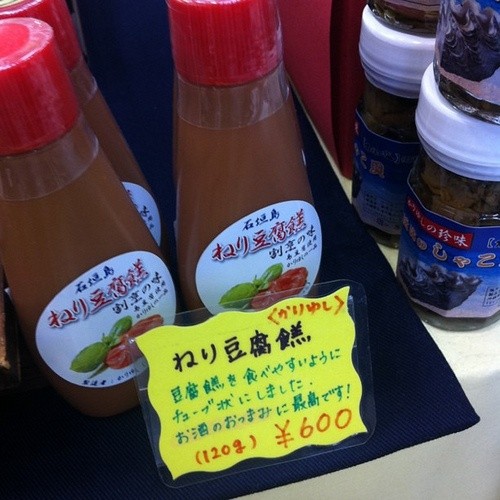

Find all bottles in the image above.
[169,1,322,324]
[1,20,186,419]
[1,0,163,255]
